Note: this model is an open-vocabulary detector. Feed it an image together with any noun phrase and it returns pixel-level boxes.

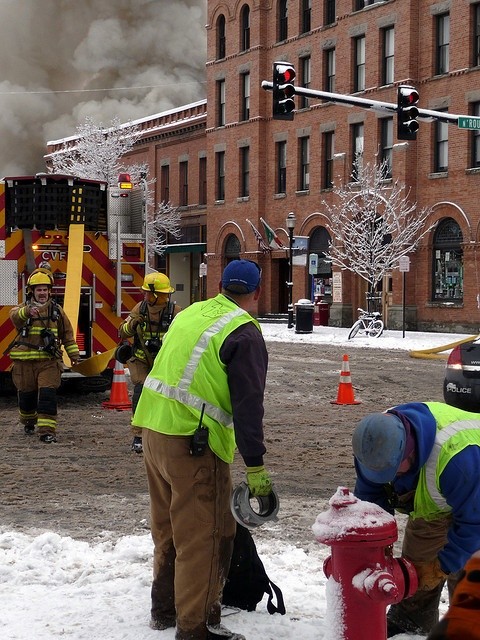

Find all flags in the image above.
[246,218,271,253]
[260,217,284,249]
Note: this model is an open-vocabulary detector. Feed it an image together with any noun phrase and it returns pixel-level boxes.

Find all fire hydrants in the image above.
[311,487,419,640]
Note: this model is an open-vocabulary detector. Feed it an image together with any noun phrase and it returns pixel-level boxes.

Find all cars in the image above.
[444,338,480,412]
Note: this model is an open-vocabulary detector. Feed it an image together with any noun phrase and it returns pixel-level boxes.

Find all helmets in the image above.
[353,413,406,484]
[141,273,175,294]
[27,268,54,286]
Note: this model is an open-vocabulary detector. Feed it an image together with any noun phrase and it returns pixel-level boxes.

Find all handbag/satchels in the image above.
[220,522,286,615]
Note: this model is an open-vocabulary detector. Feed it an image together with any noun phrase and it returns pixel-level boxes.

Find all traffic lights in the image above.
[398,87,419,140]
[273,63,296,120]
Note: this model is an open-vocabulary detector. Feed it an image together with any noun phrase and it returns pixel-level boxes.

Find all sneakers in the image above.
[24,425,35,435]
[132,438,143,454]
[149,618,175,629]
[39,434,55,442]
[387,610,439,638]
[176,626,245,640]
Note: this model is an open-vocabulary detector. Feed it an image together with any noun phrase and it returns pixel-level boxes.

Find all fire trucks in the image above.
[0,174,148,382]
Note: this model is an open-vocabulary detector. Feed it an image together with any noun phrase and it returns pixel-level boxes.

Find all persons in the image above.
[10,272,80,444]
[131,259,274,640]
[351,403,480,639]
[117,273,184,453]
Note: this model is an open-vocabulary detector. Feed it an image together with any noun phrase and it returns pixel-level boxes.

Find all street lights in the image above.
[286,212,296,328]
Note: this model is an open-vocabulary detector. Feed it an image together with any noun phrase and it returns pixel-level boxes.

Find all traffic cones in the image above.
[101,359,133,411]
[330,353,362,405]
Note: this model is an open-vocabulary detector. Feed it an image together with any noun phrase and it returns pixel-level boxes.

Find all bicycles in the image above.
[348,308,384,339]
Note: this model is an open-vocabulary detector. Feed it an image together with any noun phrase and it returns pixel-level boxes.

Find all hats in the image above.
[222,259,263,293]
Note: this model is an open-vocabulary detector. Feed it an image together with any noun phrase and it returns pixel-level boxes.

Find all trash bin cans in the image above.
[294,303,314,333]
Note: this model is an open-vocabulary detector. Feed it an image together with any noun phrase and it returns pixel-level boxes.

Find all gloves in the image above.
[415,557,449,592]
[246,465,273,497]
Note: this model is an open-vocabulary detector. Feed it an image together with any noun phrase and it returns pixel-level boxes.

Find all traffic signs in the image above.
[457,117,480,129]
